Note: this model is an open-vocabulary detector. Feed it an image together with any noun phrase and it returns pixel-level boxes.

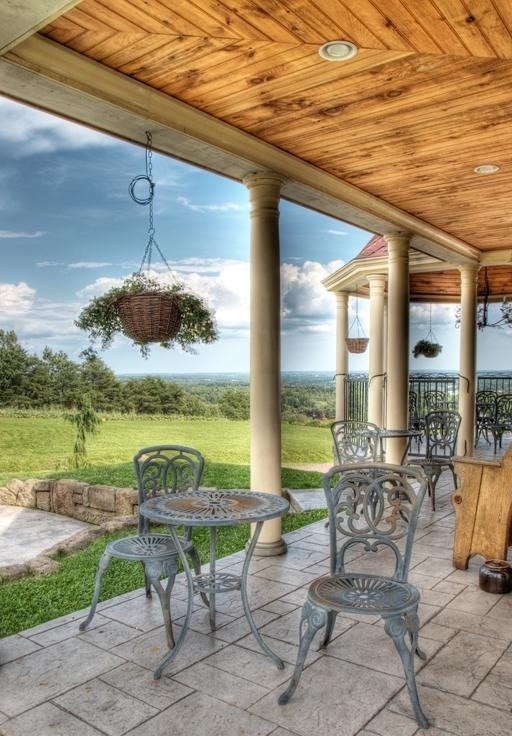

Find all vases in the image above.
[345,337,369,354]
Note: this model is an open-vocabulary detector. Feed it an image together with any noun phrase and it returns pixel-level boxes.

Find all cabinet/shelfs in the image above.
[449,439,512,571]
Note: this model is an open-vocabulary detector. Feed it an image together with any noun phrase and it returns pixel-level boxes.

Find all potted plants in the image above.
[412,338,443,359]
[73,271,221,363]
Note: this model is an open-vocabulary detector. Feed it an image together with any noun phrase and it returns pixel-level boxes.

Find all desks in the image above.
[475,403,494,448]
[139,491,290,680]
[360,430,423,524]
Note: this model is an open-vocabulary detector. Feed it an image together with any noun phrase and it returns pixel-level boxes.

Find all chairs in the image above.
[407,410,462,511]
[494,394,512,455]
[278,462,430,730]
[325,421,408,535]
[407,392,423,445]
[423,390,446,411]
[475,390,498,439]
[80,445,209,648]
